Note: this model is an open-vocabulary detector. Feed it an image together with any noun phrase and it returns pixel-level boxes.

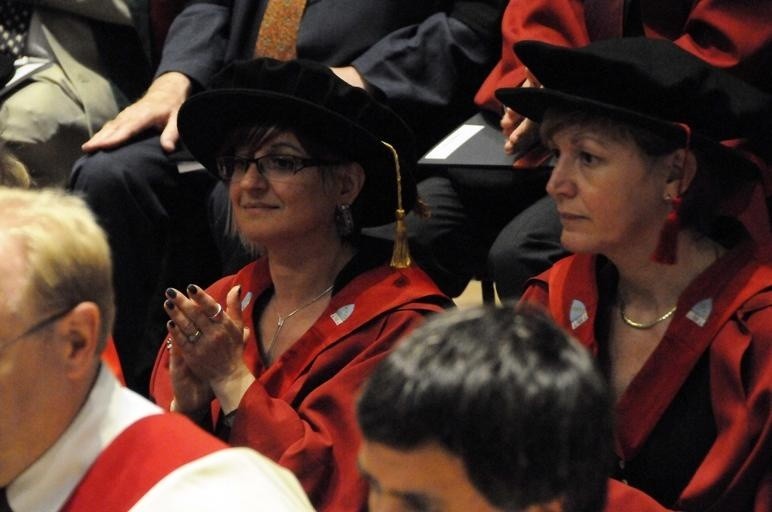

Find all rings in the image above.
[189,330,201,345]
[207,304,224,321]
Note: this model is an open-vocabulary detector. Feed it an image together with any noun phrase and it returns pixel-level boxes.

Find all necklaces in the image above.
[258,282,337,359]
[614,284,682,332]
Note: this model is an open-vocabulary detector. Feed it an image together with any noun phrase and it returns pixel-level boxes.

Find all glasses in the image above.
[217,152,321,180]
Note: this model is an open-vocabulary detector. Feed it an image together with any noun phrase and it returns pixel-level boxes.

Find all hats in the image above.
[494,36,769,180]
[177,56,415,271]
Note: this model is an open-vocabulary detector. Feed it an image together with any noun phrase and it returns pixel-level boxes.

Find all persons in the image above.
[0,186,317,510]
[499,33,771,512]
[358,301,666,512]
[147,57,461,508]
[0,0,771,401]
